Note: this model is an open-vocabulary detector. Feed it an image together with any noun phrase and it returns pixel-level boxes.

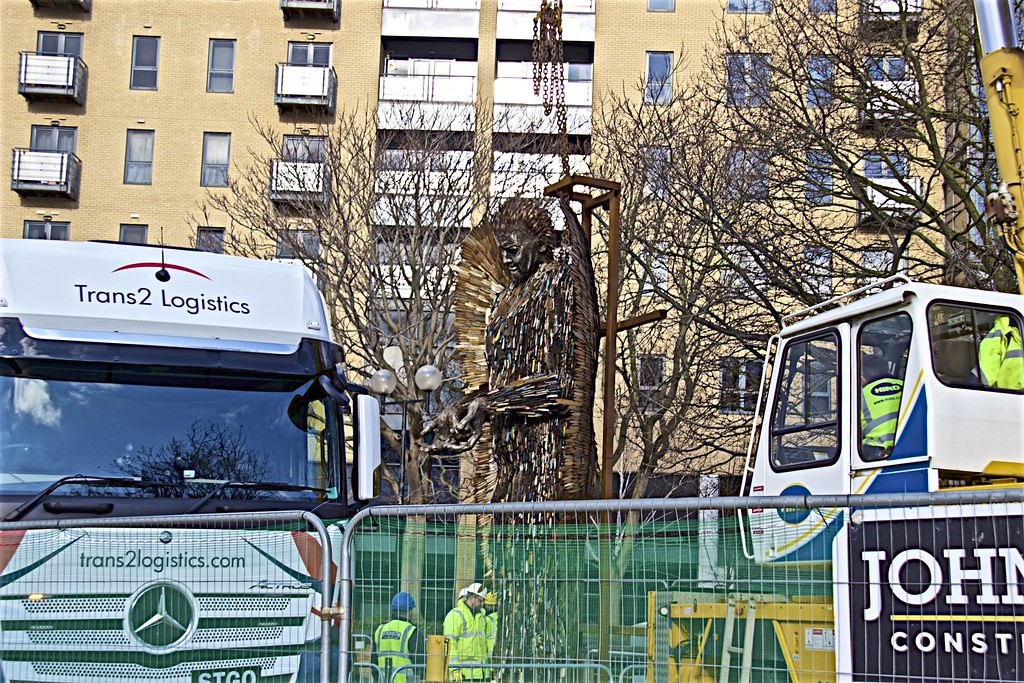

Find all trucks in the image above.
[0,236,380,683]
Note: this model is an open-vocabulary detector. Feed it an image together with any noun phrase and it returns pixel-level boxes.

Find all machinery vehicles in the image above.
[737,0,1024,571]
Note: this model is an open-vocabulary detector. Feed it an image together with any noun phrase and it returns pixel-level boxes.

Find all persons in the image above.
[371,592,426,683]
[861,354,903,461]
[443,583,494,683]
[418,196,601,683]
[458,587,486,616]
[484,592,498,662]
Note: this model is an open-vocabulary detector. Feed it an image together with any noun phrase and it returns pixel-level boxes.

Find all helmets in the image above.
[391,592,416,611]
[458,588,468,599]
[467,583,488,599]
[483,591,498,605]
